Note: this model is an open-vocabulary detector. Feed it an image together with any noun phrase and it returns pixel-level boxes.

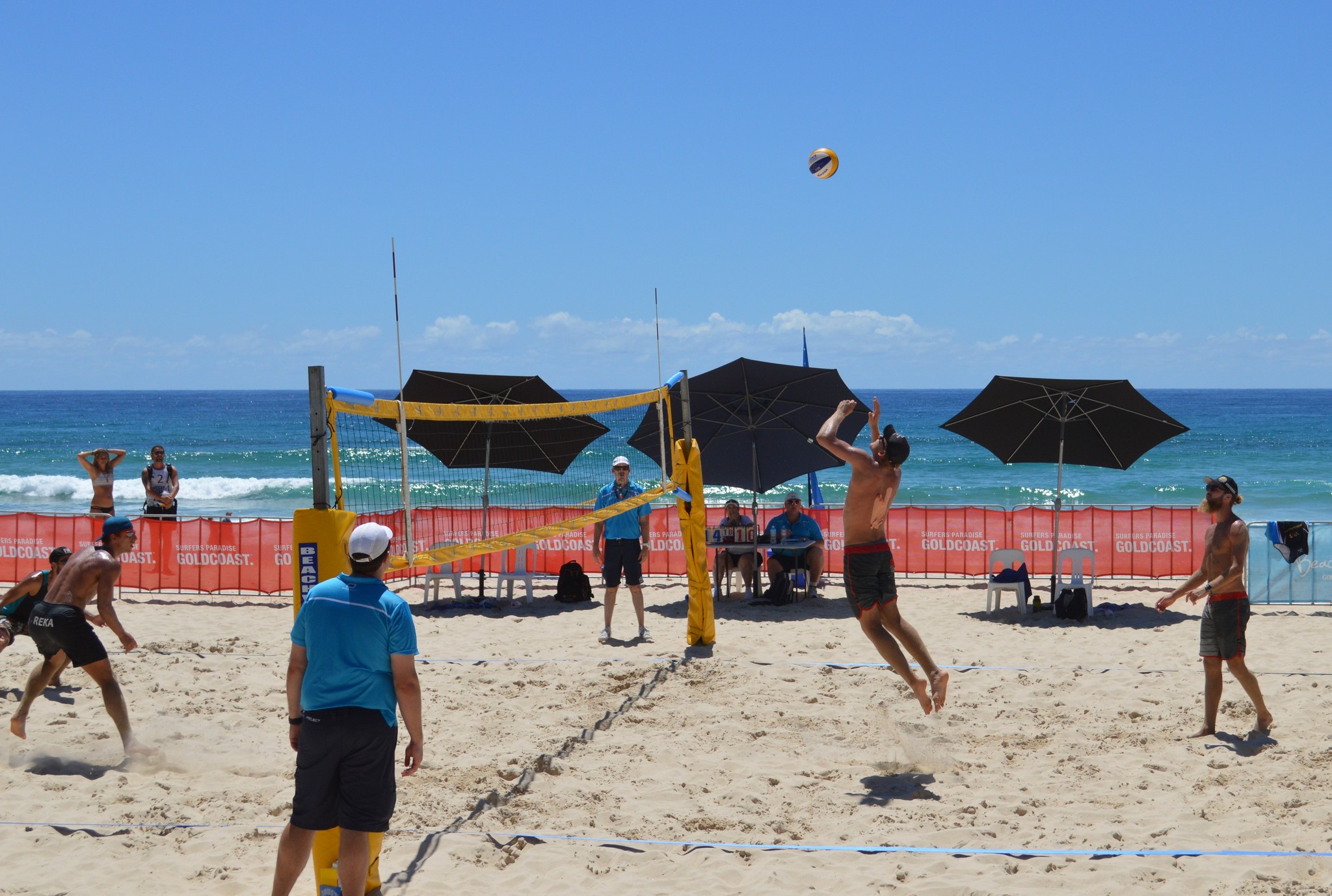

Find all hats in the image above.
[883,424,910,465]
[94,516,134,542]
[226,511,232,515]
[1203,475,1238,499]
[612,456,629,465]
[349,522,393,562]
[49,546,74,562]
[784,492,801,501]
[725,499,740,509]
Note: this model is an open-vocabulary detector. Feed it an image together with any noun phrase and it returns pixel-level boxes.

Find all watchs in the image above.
[1205,584,1213,595]
[642,543,650,548]
[739,522,741,525]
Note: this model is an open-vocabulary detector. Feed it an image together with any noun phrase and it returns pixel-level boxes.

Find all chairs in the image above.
[786,568,810,594]
[727,565,762,597]
[497,542,537,603]
[1053,548,1094,617]
[423,542,462,605]
[986,549,1028,615]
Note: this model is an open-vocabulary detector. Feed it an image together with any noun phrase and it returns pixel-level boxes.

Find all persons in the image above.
[77,448,126,520]
[592,456,652,641]
[759,492,824,605]
[141,445,179,521]
[10,516,160,756]
[711,499,763,603]
[0,546,108,654]
[1153,475,1273,738]
[271,522,423,896]
[816,397,949,715]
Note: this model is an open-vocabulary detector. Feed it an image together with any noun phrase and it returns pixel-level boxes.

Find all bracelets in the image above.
[288,716,303,725]
[736,520,741,525]
[91,451,94,455]
[106,449,109,452]
[727,519,735,525]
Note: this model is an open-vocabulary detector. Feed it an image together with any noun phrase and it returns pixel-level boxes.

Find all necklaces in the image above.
[100,468,108,482]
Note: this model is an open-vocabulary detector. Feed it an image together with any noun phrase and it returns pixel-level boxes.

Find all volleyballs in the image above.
[156,490,170,506]
[808,148,838,179]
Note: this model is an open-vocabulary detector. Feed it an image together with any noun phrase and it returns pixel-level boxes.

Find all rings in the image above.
[646,559,647,560]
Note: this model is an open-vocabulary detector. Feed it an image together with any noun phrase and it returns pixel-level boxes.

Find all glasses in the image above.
[785,500,801,505]
[151,451,165,454]
[725,500,738,504]
[116,530,136,537]
[56,559,68,566]
[1206,483,1228,492]
[612,466,629,471]
[879,436,886,449]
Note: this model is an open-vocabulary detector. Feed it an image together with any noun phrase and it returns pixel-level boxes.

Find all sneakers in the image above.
[638,627,653,640]
[598,627,612,641]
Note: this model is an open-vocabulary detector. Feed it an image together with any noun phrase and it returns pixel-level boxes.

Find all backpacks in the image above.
[1054,587,1087,620]
[763,572,796,606]
[554,559,595,602]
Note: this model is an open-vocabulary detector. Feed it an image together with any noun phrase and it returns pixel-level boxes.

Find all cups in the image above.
[1104,608,1114,617]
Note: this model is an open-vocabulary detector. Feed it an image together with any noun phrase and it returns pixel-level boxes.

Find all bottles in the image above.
[479,596,495,607]
[483,602,501,611]
[781,526,786,544]
[511,601,521,609]
[770,526,776,545]
[1032,596,1041,613]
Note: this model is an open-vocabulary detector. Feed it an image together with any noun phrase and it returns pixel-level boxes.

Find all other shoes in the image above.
[744,587,752,599]
[711,588,723,598]
[808,587,819,598]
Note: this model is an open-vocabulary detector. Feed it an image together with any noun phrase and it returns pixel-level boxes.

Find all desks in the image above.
[705,538,815,603]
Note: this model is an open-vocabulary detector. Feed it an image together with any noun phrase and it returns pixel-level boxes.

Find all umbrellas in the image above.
[626,357,873,599]
[938,375,1191,604]
[371,369,611,602]
